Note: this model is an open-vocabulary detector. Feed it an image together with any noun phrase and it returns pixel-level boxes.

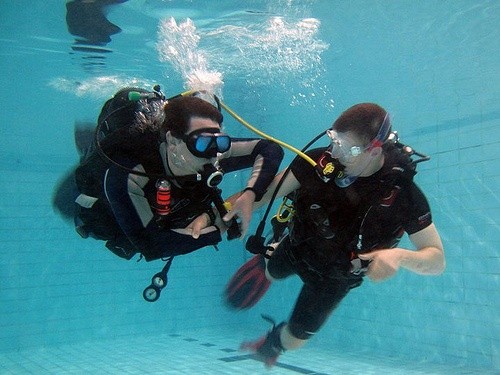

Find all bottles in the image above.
[157,179,171,215]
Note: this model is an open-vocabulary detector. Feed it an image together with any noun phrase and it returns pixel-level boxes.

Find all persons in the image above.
[51,90,285,259]
[185,105,446,370]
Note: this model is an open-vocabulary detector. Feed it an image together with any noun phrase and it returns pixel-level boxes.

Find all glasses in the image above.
[326,130,352,153]
[173,127,231,159]
